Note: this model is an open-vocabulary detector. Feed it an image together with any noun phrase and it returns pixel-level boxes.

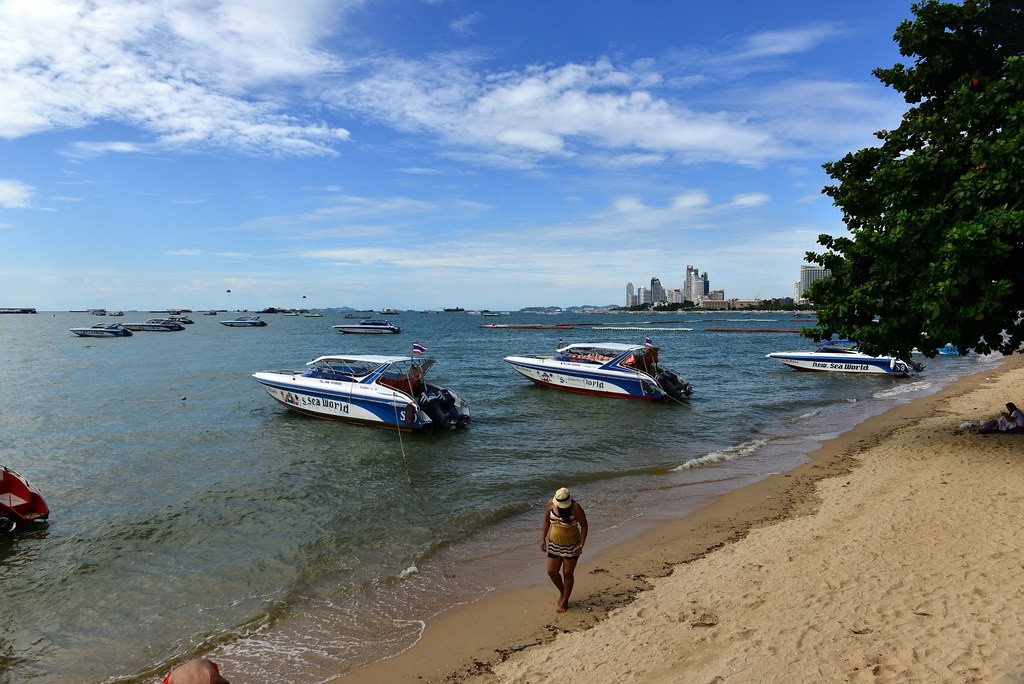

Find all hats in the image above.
[552,487,571,508]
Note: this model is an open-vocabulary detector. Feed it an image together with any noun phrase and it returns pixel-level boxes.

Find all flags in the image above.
[560,337,564,344]
[646,336,653,349]
[412,341,428,355]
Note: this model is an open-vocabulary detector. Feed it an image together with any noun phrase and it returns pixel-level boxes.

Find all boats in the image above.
[251,354,473,435]
[332,318,403,334]
[909,341,960,356]
[503,342,697,409]
[219,315,268,327]
[443,306,511,316]
[764,333,927,380]
[380,308,400,314]
[69,322,133,338]
[89,307,217,332]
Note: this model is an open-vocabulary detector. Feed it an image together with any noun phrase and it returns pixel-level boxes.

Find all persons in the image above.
[998,402,1024,430]
[541,487,588,613]
[162,659,229,684]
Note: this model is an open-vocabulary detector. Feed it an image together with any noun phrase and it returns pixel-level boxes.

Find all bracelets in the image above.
[580,544,583,548]
